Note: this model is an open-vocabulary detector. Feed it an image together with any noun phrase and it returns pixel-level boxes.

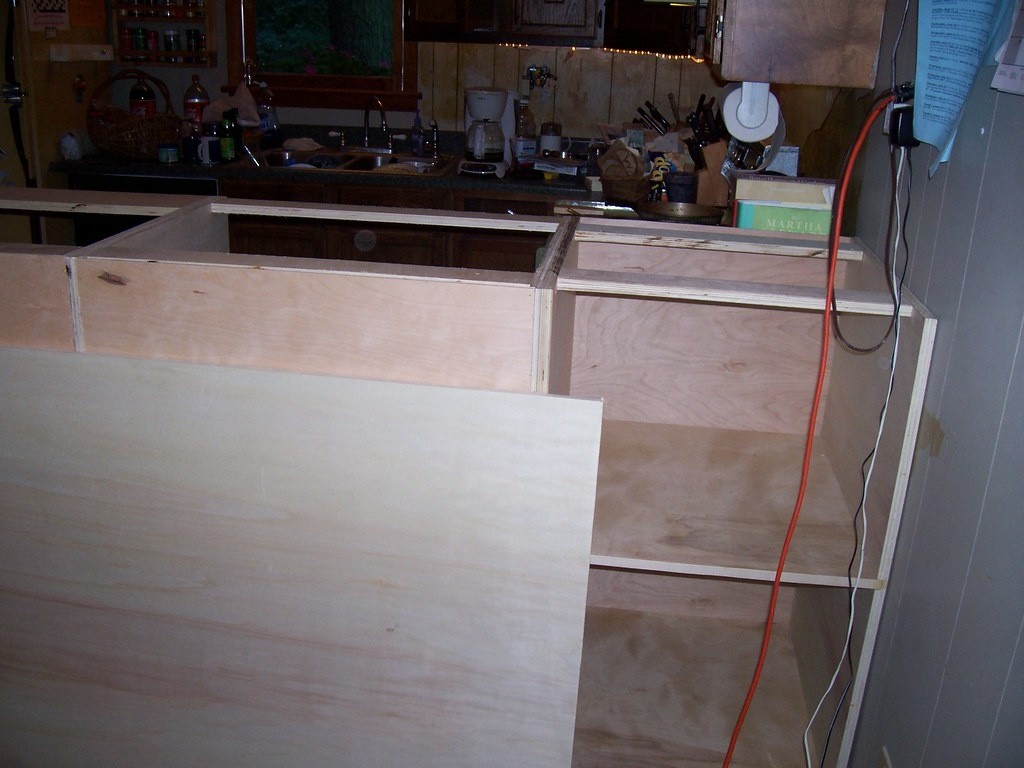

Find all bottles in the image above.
[183,75,210,124]
[129,78,156,116]
[220,111,236,161]
[118,0,205,63]
[512,98,537,179]
[256,83,280,132]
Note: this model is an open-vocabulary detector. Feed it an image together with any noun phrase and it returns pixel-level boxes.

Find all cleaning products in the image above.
[255,81,279,147]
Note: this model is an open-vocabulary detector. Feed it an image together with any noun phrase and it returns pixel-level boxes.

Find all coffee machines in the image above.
[456,86,510,179]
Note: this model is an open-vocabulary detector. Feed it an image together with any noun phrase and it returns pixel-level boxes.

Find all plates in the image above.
[373,163,417,174]
[406,161,427,173]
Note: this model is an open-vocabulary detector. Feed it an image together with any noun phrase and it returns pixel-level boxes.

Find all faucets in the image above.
[361,93,388,151]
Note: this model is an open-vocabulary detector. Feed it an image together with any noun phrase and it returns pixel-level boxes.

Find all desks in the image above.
[0,344,606,768]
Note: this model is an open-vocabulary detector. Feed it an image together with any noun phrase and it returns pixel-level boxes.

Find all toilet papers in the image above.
[720,84,786,176]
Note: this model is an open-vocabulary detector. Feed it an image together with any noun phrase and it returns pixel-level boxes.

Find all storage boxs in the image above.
[759,137,800,177]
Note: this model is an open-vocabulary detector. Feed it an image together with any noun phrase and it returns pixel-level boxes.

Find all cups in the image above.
[197,136,220,164]
[539,122,571,159]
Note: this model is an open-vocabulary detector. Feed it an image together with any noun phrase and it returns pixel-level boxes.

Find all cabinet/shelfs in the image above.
[405,0,887,89]
[534,215,938,768]
[110,0,218,69]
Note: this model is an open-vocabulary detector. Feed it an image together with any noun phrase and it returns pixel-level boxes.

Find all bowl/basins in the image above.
[639,201,725,226]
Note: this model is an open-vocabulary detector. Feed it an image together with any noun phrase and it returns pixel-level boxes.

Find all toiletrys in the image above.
[410,108,425,156]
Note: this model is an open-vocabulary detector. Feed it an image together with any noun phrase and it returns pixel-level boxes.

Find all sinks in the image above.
[242,141,360,171]
[336,152,459,176]
